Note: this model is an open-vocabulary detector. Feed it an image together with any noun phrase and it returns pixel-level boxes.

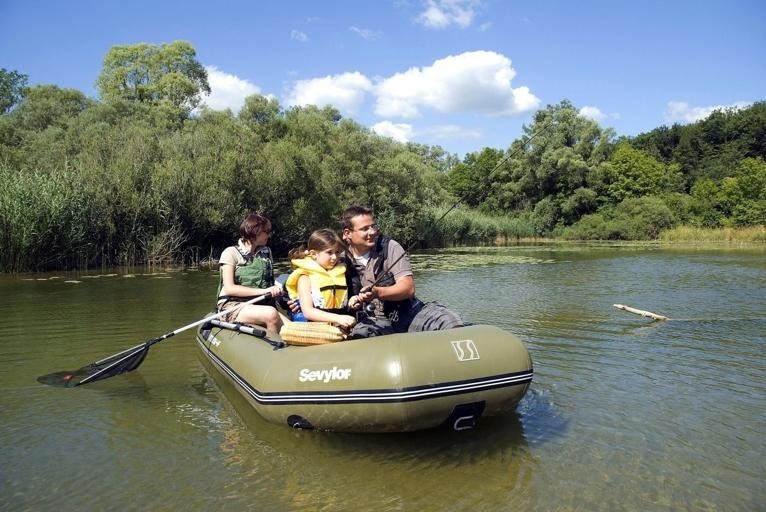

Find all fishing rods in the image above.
[353,123,551,309]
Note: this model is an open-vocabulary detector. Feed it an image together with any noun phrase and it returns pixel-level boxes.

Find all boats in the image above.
[195,299,536,431]
[189,348,282,451]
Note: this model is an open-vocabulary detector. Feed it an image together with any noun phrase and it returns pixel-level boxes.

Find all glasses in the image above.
[347,224,379,232]
[263,230,270,236]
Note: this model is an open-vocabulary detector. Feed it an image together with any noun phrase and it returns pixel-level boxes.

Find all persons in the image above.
[214,213,284,339]
[286,205,464,338]
[285,227,360,339]
